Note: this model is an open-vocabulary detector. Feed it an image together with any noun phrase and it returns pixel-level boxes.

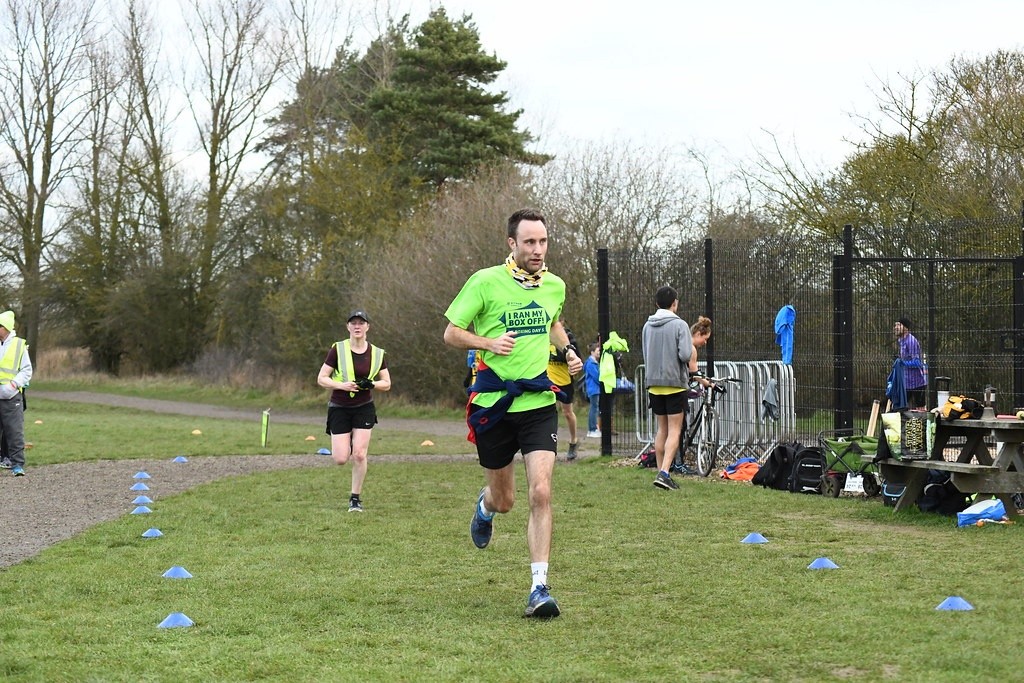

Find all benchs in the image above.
[858,454,1000,477]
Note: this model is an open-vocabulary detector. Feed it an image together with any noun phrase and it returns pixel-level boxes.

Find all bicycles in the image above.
[678,369,743,478]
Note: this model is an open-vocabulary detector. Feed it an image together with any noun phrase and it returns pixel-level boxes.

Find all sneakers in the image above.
[669,464,674,472]
[470,486,495,549]
[11,466,24,475]
[522,580,560,618]
[348,496,362,512]
[673,465,697,475]
[349,432,354,455]
[653,472,680,490]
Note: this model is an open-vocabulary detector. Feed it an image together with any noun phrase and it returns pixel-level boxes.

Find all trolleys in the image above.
[816,404,880,497]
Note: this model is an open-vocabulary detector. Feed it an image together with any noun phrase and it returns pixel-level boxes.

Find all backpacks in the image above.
[789,444,827,493]
[764,445,797,491]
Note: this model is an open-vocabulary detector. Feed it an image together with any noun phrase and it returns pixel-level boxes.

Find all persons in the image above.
[641,286,693,491]
[668,316,715,475]
[548,314,582,461]
[315,311,391,513]
[0,310,33,477]
[584,342,600,438]
[444,208,584,618]
[895,318,927,413]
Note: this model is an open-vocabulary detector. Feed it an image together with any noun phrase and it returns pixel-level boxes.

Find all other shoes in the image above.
[0,458,12,468]
[587,430,601,437]
[611,430,618,435]
[566,441,578,459]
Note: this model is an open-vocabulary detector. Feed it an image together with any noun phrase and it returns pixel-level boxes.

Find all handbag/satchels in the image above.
[881,409,936,461]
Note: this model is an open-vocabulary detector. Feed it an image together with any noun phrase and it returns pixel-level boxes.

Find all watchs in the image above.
[562,344,577,358]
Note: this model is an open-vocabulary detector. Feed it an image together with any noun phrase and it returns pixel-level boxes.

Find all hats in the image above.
[895,318,910,330]
[0,311,15,332]
[347,309,369,322]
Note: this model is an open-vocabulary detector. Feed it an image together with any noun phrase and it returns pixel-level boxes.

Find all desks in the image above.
[893,412,1024,520]
